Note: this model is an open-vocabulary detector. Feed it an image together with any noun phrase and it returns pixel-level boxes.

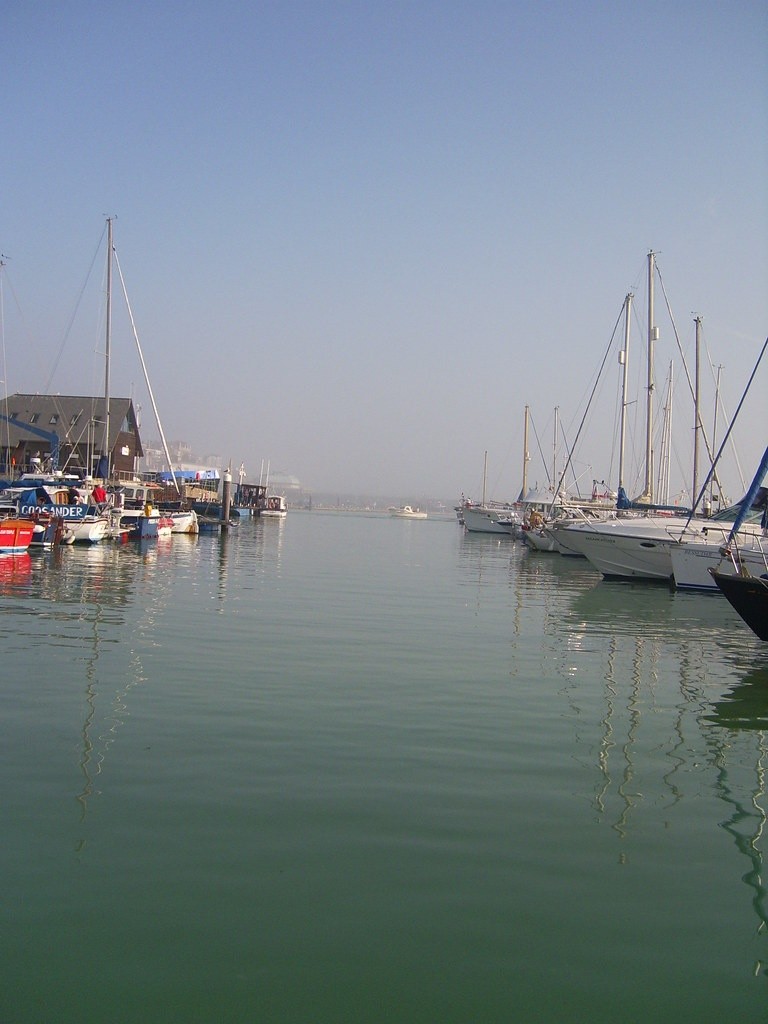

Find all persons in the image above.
[68,485,80,504]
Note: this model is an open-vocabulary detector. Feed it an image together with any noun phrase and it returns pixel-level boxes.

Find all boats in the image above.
[260,496,288,518]
[0,209,271,555]
[387,505,427,520]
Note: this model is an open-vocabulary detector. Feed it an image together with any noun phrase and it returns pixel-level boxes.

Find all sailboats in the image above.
[452,247,767,644]
